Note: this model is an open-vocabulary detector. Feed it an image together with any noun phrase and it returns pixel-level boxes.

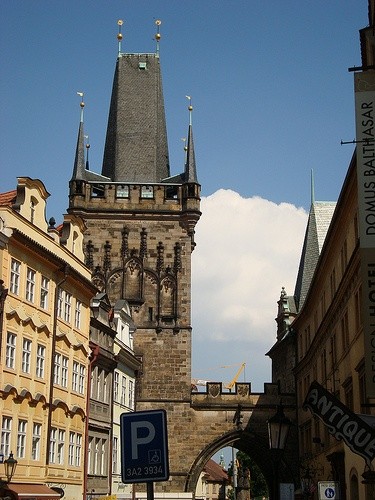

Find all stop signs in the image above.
[120,410,169,485]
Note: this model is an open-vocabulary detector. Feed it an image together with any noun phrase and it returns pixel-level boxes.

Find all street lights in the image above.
[5,452,18,483]
